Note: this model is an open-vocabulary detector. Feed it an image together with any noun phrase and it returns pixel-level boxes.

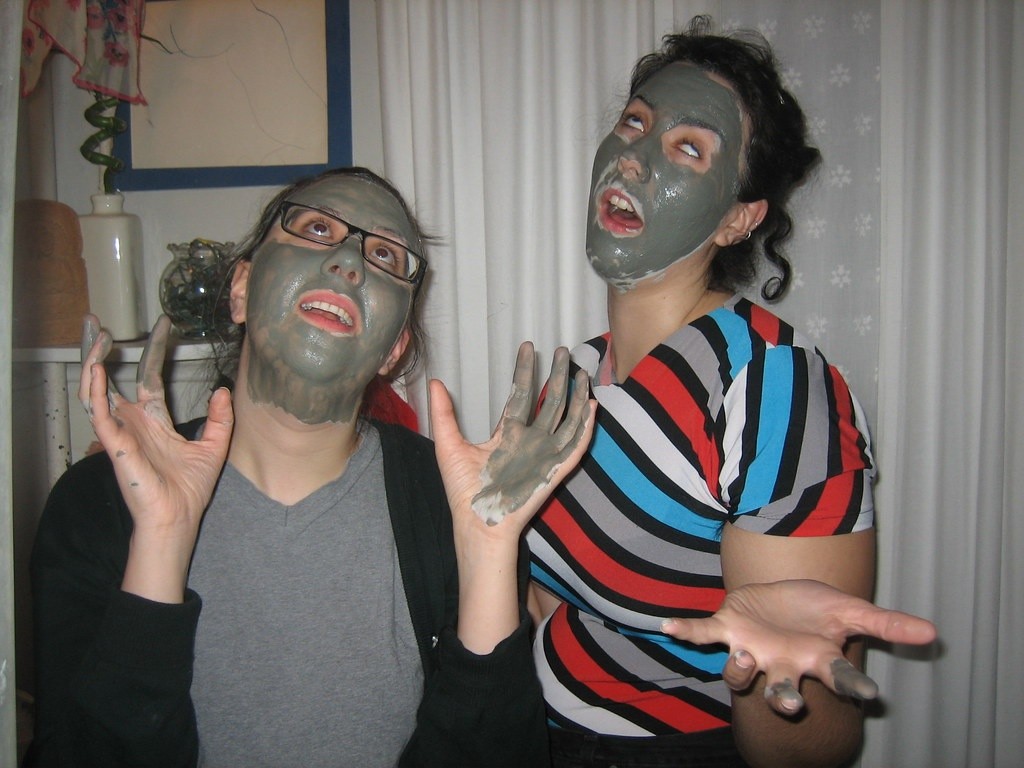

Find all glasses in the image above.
[246,201,428,309]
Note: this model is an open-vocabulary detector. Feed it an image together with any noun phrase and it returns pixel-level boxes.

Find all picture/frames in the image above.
[103,0,352,191]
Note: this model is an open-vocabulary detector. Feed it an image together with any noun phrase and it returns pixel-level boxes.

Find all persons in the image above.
[22,168,596,768]
[520,29,938,767]
